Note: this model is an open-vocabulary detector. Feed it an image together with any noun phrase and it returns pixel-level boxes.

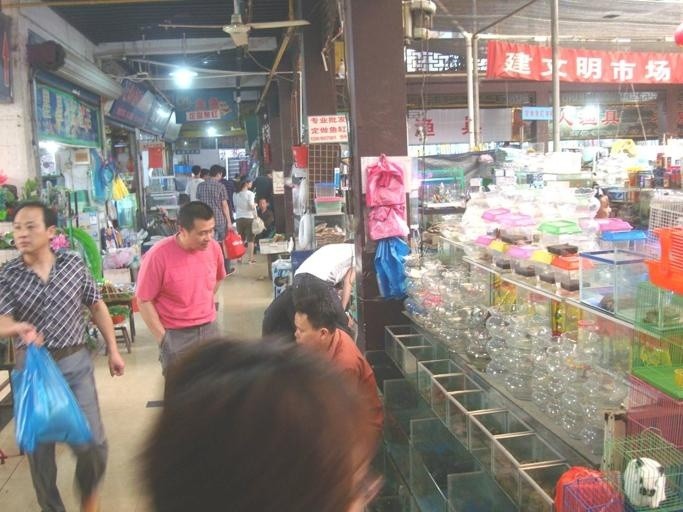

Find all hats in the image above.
[240,177,256,184]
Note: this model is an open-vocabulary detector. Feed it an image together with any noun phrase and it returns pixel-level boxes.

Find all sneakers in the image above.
[249,259,256,264]
[227,268,235,277]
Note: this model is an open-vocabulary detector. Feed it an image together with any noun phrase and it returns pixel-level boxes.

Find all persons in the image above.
[184,165,276,278]
[143,336,384,512]
[293,239,357,342]
[2,201,125,512]
[293,295,385,454]
[262,283,298,343]
[136,201,225,379]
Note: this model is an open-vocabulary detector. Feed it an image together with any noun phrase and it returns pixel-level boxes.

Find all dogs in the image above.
[625,457,666,508]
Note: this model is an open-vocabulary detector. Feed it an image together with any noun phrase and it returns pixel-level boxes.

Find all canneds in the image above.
[623,152,683,202]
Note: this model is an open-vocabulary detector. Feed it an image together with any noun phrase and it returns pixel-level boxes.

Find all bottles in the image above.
[653,152,683,191]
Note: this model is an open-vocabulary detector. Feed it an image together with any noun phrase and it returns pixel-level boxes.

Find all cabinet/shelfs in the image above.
[364,236,683,512]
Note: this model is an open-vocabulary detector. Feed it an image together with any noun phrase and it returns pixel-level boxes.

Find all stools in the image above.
[105,326,132,354]
[107,299,135,336]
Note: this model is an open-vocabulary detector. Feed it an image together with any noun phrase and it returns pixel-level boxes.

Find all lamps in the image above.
[168,32,199,89]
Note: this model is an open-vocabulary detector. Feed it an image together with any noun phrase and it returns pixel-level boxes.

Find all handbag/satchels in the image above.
[223,230,246,259]
[252,217,265,235]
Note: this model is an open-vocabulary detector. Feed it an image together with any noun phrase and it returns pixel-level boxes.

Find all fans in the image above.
[157,0,310,46]
